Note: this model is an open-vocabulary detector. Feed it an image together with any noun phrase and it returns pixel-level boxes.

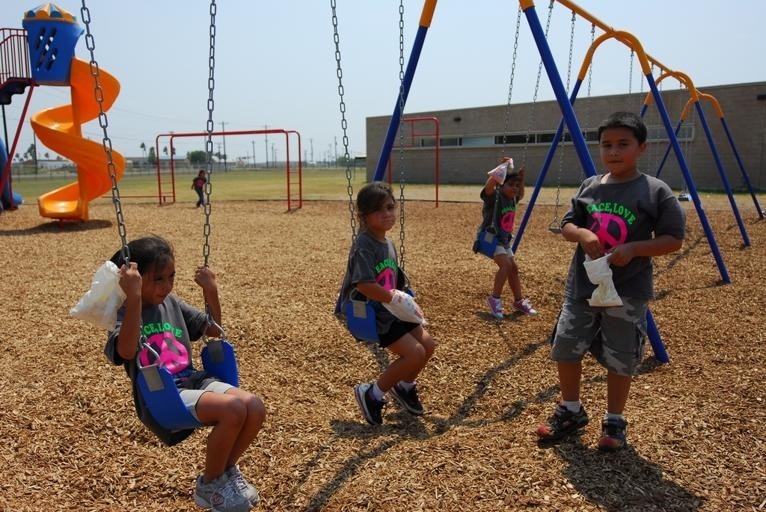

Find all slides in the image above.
[30,54,125,220]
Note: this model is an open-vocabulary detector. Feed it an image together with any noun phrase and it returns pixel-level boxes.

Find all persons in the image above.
[335,183,435,426]
[537,111,686,452]
[191,170,207,207]
[472,156,538,320]
[110,237,266,512]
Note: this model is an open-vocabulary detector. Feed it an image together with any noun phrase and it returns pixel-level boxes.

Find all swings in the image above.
[331,1,414,342]
[81,1,238,432]
[679,80,697,202]
[479,0,556,257]
[549,2,594,233]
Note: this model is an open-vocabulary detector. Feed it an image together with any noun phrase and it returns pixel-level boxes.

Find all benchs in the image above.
[102,196,176,204]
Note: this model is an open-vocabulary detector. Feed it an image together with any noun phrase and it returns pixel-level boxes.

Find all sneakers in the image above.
[512,299,538,317]
[193,472,251,512]
[354,381,388,425]
[226,464,260,505]
[538,404,589,439]
[487,296,504,319]
[599,417,629,451]
[389,382,423,414]
